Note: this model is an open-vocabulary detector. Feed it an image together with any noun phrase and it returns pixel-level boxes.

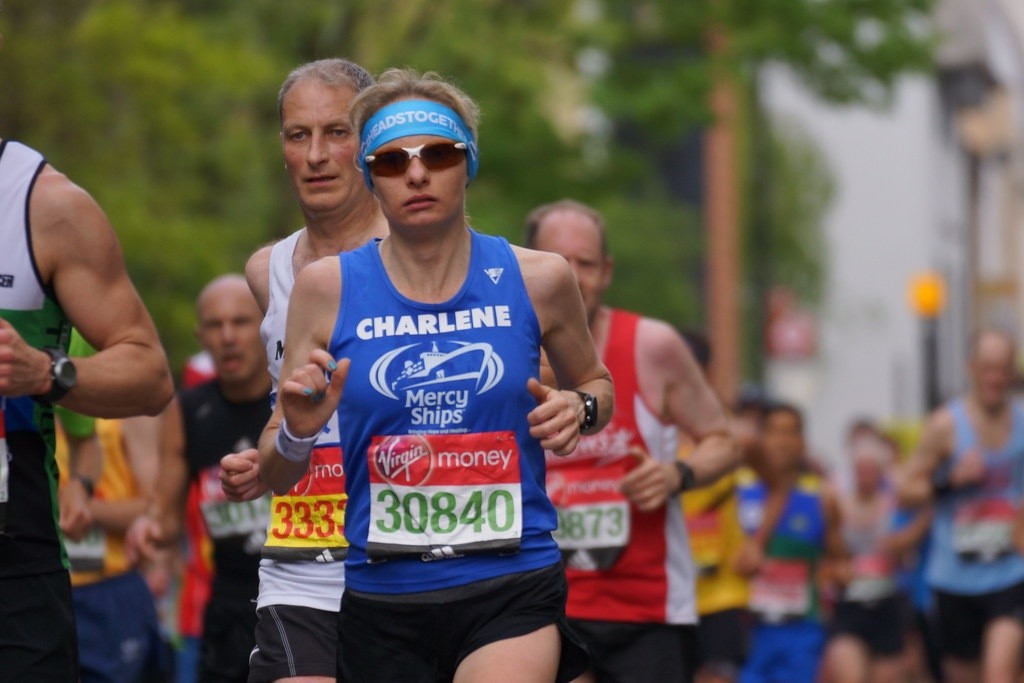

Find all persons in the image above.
[528,201,741,683]
[0,33,175,683]
[673,324,1024,682]
[55,274,272,682]
[258,69,613,682]
[219,59,389,682]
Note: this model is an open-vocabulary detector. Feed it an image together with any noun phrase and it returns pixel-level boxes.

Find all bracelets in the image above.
[75,473,95,496]
[676,459,695,490]
[275,420,322,462]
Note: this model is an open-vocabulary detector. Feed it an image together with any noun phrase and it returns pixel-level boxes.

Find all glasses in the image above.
[365,142,468,177]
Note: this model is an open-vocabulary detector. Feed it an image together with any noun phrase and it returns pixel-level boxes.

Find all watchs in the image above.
[573,389,598,430]
[34,348,77,407]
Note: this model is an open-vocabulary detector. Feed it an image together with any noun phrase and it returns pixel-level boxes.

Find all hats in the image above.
[735,386,771,411]
